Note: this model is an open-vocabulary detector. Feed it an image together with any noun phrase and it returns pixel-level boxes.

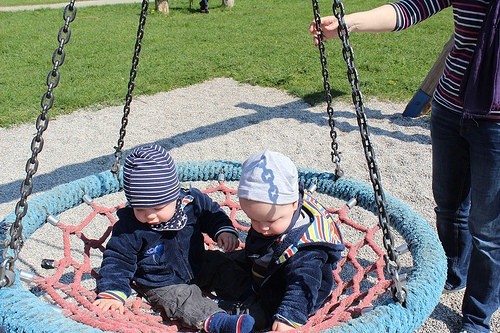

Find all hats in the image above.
[237,150,301,206]
[122,145,183,209]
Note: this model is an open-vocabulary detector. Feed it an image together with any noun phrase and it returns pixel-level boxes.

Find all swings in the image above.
[0,0,449,333]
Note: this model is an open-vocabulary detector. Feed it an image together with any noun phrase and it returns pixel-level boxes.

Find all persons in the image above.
[88,144,255,333]
[310,0,500,333]
[205,150,348,332]
